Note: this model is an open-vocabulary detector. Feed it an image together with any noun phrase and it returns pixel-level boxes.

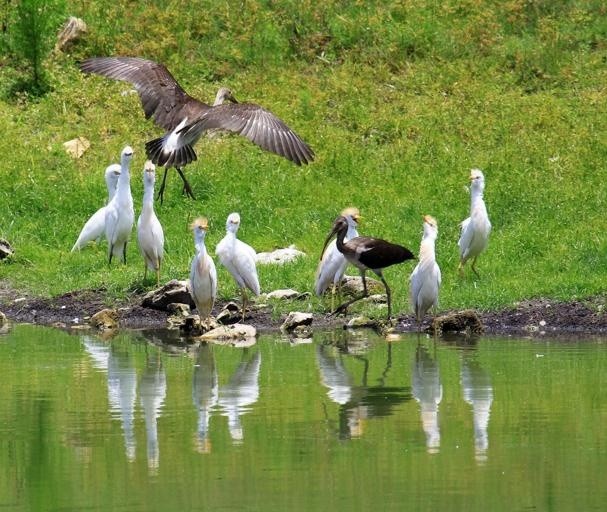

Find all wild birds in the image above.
[69,162,122,255]
[313,206,367,315]
[319,214,417,323]
[74,54,317,209]
[136,158,165,290]
[315,337,363,440]
[217,344,263,442]
[139,339,168,473]
[408,212,442,329]
[105,145,135,267]
[412,329,444,456]
[215,211,261,324]
[105,334,139,464]
[458,348,495,462]
[185,215,219,335]
[189,341,219,455]
[337,331,414,446]
[458,166,493,280]
